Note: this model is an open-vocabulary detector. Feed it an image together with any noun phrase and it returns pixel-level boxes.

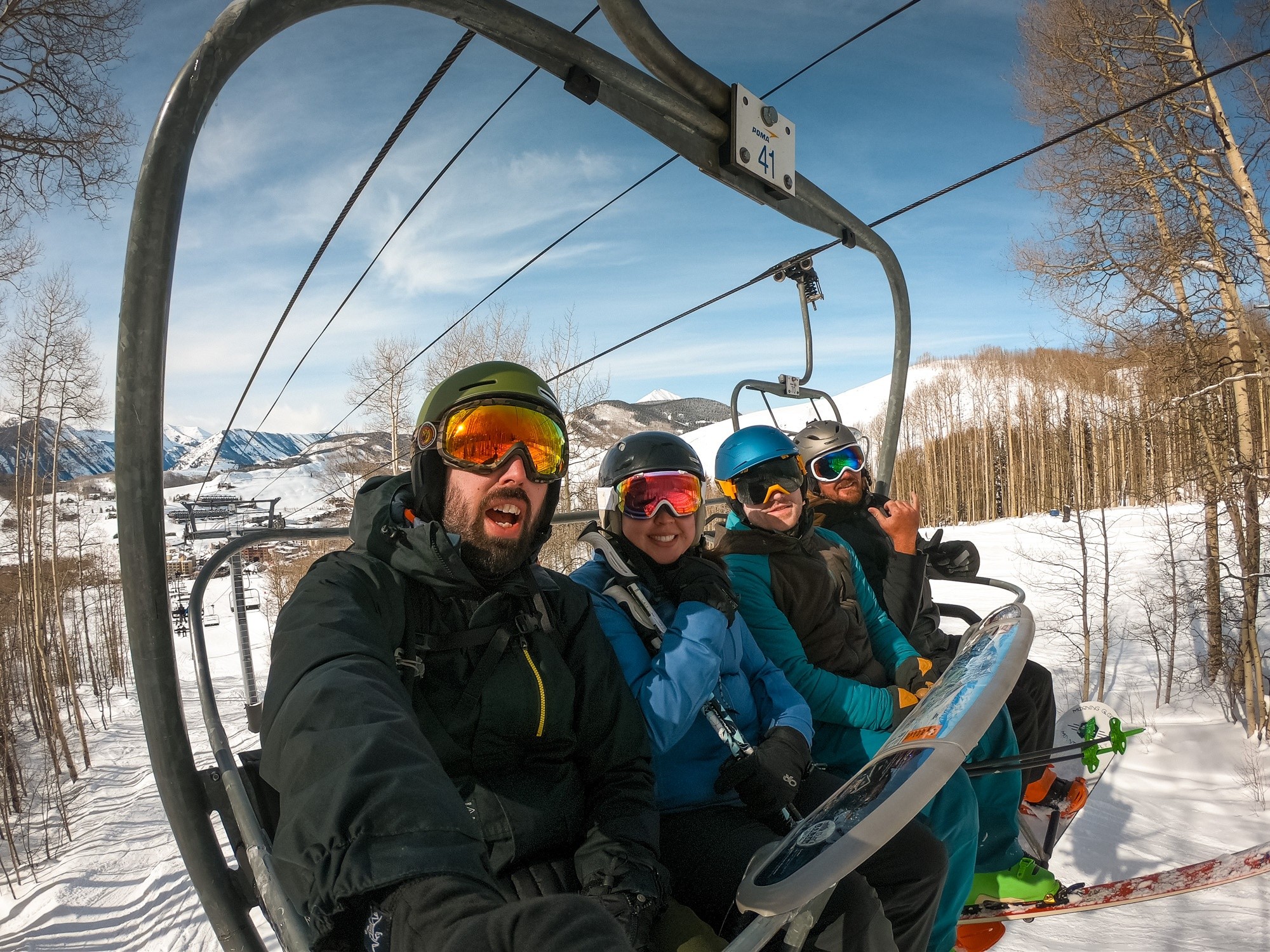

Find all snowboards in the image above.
[1012,699,1123,865]
[955,839,1270,926]
[954,920,1006,952]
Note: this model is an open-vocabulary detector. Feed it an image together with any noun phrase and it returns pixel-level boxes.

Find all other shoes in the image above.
[954,916,1005,952]
[958,855,1061,916]
[1016,763,1088,819]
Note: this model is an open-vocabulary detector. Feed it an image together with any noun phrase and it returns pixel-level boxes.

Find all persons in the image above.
[178,604,188,623]
[566,431,949,952]
[793,421,1088,819]
[177,625,187,636]
[710,425,1060,951]
[253,362,730,952]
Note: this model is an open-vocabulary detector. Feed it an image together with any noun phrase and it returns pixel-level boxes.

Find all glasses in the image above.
[732,454,803,505]
[435,397,569,484]
[616,471,702,521]
[811,444,865,482]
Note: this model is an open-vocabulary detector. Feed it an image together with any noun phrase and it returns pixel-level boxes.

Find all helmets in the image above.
[595,430,705,488]
[409,360,569,461]
[794,418,858,466]
[715,425,802,481]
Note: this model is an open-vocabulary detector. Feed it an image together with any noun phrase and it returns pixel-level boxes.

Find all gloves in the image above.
[887,682,929,731]
[586,876,653,950]
[931,541,980,581]
[676,545,741,630]
[714,726,811,828]
[895,657,939,698]
